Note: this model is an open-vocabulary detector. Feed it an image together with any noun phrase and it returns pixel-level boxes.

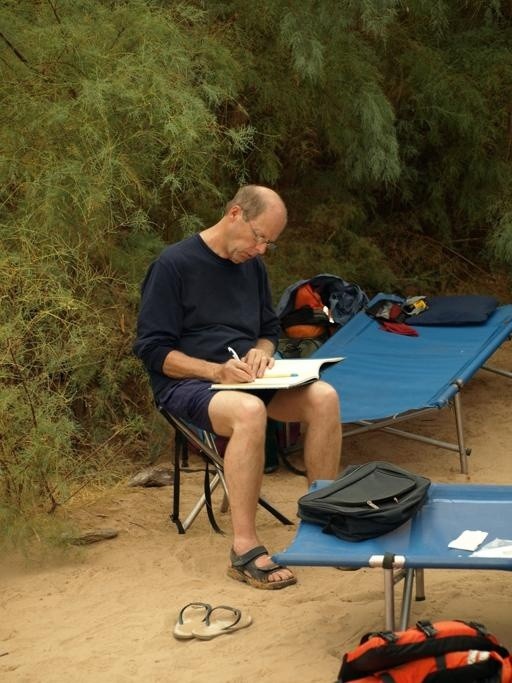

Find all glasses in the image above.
[239,203,276,250]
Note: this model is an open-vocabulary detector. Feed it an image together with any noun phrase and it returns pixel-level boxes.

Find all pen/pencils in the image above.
[228,346,255,381]
[256,373,298,377]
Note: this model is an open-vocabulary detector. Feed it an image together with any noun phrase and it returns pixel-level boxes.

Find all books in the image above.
[207,357,346,391]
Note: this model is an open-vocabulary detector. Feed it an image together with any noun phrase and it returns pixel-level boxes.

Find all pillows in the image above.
[405,296,499,325]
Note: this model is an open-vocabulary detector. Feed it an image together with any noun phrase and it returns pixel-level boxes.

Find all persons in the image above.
[131,185,361,590]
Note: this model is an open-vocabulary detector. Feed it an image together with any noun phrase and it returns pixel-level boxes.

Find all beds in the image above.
[271,479,512,632]
[283,293,512,475]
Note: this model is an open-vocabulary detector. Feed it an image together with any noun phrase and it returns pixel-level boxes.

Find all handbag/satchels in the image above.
[275,273,369,358]
[336,618,512,682]
[297,460,431,542]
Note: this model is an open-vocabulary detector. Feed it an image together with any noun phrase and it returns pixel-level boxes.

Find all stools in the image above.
[154,398,294,533]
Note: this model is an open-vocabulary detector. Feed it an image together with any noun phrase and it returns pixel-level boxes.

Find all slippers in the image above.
[174,600,210,640]
[192,605,250,640]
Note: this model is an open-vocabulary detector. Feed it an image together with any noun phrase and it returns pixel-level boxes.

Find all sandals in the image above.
[226,546,296,589]
